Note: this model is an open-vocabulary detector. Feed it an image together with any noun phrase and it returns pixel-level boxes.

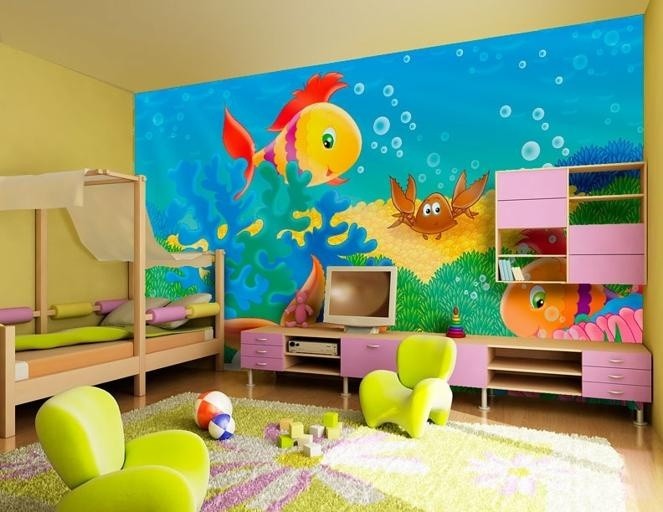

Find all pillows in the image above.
[155,293,212,329]
[100,298,171,327]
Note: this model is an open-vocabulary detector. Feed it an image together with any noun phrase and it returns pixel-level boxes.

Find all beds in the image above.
[1,168,225,439]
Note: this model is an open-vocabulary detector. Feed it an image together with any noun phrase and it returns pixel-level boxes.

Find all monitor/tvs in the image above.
[323,266,398,335]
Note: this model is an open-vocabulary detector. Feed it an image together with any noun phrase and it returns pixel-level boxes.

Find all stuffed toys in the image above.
[284,293,314,329]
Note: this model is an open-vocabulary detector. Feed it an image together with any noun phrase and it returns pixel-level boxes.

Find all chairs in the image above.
[35,385,209,512]
[359,333,458,437]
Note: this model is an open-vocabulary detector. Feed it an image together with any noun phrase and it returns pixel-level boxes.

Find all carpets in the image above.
[1,392,627,512]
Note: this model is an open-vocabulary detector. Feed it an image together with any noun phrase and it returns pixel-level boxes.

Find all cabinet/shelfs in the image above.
[240,327,652,427]
[495,162,647,286]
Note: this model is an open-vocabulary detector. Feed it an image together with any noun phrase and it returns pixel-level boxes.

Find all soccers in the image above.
[194,390,232,428]
[208,415,235,441]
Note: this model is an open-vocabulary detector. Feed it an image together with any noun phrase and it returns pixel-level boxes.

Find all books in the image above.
[497,259,525,281]
